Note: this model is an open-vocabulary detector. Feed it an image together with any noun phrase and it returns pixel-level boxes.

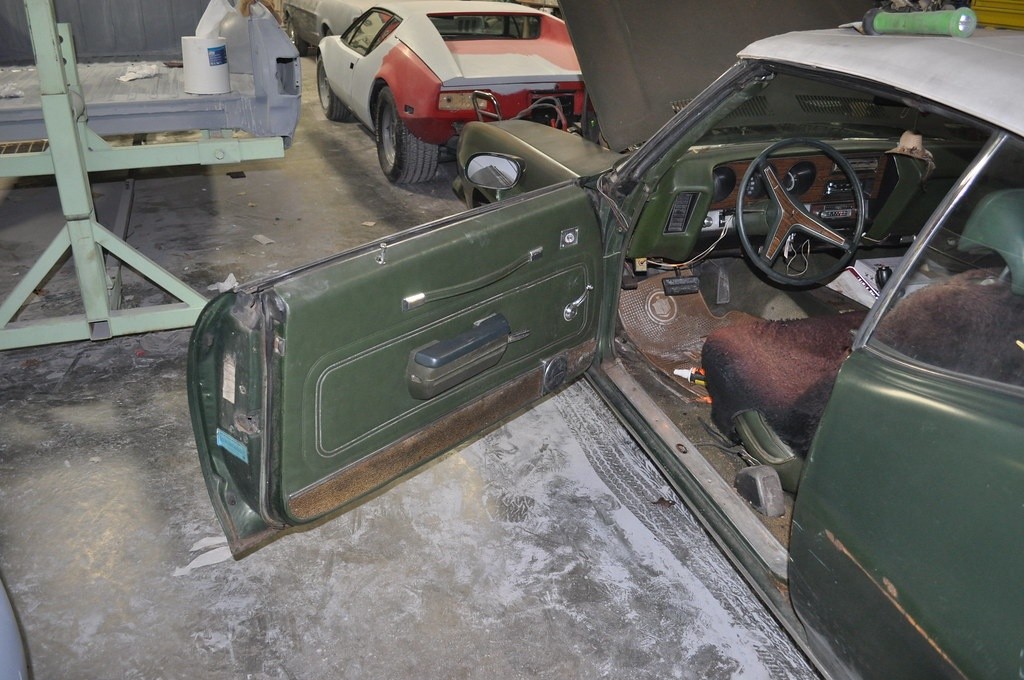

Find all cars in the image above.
[317,1,599,187]
[282,0,376,60]
[182,10,1024,680]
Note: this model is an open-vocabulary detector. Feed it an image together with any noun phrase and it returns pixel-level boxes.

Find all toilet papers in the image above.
[181,0,237,94]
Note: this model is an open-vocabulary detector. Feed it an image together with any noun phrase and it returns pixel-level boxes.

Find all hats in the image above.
[884,131,933,163]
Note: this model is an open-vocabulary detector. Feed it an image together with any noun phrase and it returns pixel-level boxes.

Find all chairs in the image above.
[702,187,1024,494]
